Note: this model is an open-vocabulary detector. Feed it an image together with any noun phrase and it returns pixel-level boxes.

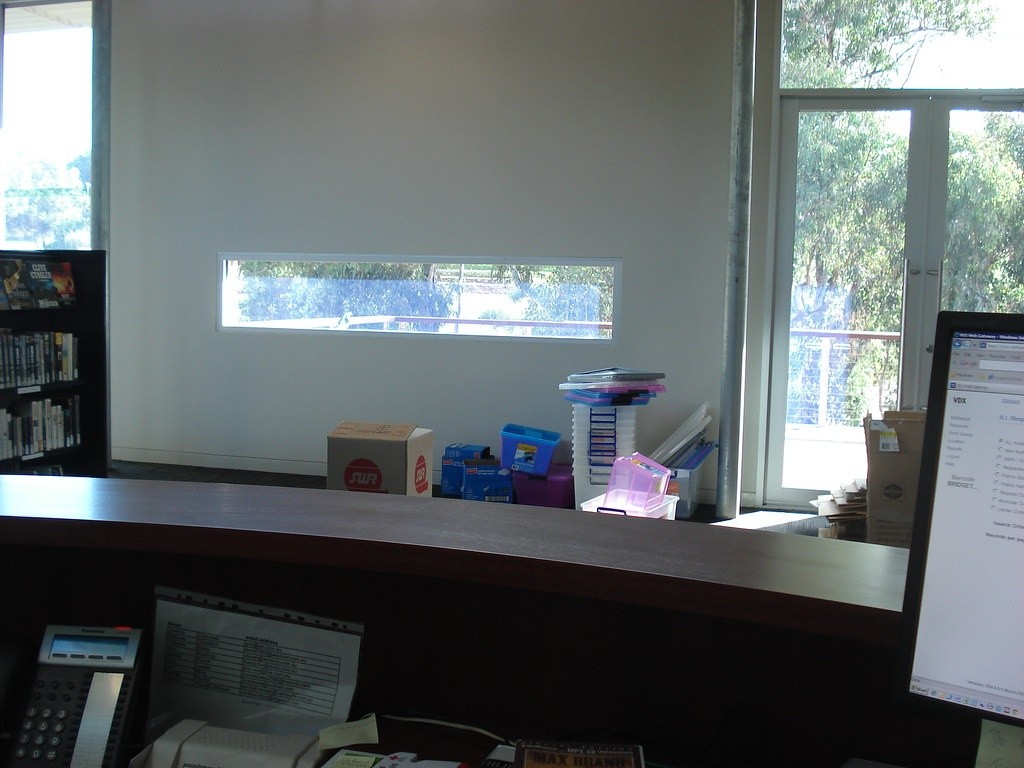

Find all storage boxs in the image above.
[326,422,434,498]
[863,411,925,549]
[440,401,719,520]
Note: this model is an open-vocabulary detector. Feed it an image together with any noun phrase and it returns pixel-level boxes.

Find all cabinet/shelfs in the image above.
[1,249,108,477]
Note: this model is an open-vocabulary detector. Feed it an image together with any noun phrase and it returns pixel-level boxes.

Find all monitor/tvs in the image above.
[889,312,1024,727]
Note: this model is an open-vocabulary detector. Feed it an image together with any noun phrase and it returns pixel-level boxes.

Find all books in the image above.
[0,395,80,460]
[28,464,63,476]
[0,327,79,387]
[476,739,644,768]
[0,257,78,309]
